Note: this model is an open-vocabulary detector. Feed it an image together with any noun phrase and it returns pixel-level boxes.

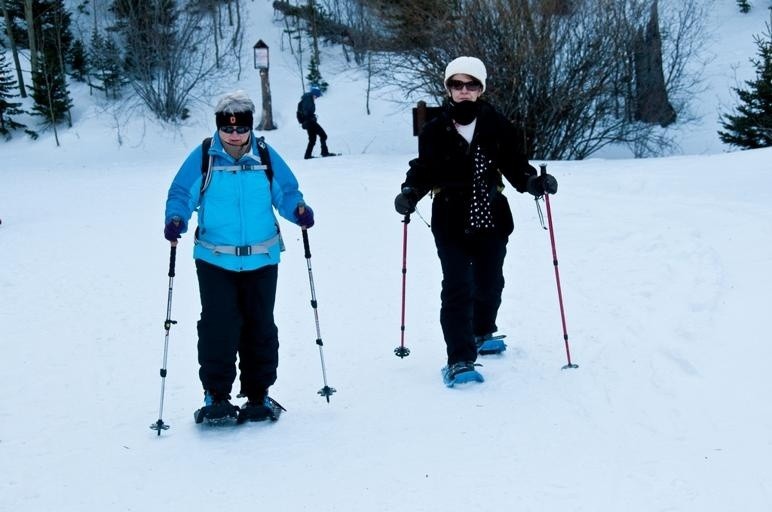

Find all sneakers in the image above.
[443,361,485,387]
[304,153,336,159]
[476,334,506,361]
[239,395,281,423]
[205,392,236,424]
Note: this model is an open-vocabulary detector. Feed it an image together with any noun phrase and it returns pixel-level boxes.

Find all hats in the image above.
[311,86,321,97]
[443,55,488,94]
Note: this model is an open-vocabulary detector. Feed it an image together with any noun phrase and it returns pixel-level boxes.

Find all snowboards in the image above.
[301,152,342,160]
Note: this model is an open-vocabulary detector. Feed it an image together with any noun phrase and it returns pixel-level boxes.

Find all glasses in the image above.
[447,79,481,92]
[220,126,249,134]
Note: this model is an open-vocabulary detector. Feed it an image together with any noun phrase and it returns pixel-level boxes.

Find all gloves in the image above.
[164,221,183,241]
[297,207,315,229]
[395,189,415,214]
[528,173,558,196]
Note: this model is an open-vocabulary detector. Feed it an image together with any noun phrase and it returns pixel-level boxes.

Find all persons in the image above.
[163,90,315,427]
[296,88,338,160]
[395,56,558,388]
